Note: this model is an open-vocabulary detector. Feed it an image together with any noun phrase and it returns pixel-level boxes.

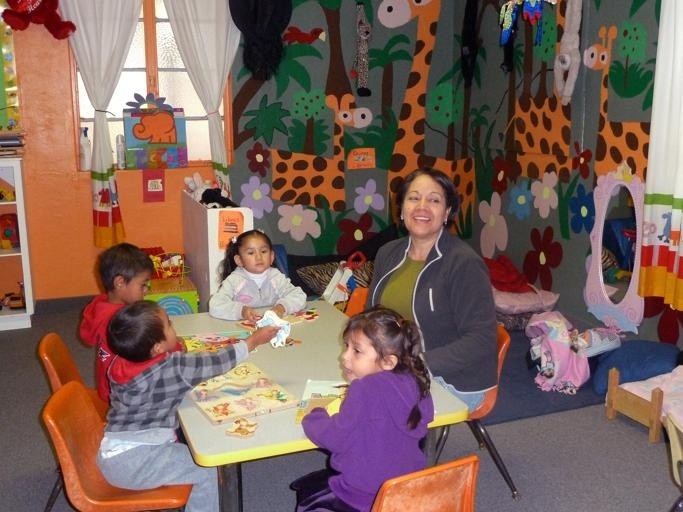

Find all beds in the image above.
[603,362,683,443]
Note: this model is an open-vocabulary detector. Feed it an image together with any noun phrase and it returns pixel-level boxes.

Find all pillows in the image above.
[595,336,677,394]
[352,222,399,261]
[294,258,375,299]
[489,283,560,316]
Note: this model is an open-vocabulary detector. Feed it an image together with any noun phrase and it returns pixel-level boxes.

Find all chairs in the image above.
[369,457,481,511]
[428,320,521,497]
[36,332,114,430]
[42,381,194,510]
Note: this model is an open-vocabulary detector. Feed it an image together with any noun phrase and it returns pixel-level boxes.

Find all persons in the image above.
[96,300,281,512]
[366,167,499,416]
[209,230,307,321]
[294,304,434,512]
[79,242,188,402]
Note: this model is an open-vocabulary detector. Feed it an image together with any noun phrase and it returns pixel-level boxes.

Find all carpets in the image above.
[474,326,605,426]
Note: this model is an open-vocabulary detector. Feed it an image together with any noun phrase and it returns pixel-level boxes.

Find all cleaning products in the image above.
[78,126,92,172]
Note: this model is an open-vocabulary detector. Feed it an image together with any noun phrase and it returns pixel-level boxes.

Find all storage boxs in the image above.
[142,276,200,316]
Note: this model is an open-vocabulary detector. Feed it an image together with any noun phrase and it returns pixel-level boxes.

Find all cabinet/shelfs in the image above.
[178,188,254,312]
[0,157,38,332]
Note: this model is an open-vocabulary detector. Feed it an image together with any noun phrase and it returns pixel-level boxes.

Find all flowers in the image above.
[238,140,388,255]
[476,140,598,293]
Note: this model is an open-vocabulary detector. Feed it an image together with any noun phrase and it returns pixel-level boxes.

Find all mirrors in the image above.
[582,162,648,336]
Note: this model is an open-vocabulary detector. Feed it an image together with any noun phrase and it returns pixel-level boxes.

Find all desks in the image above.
[157,295,470,511]
